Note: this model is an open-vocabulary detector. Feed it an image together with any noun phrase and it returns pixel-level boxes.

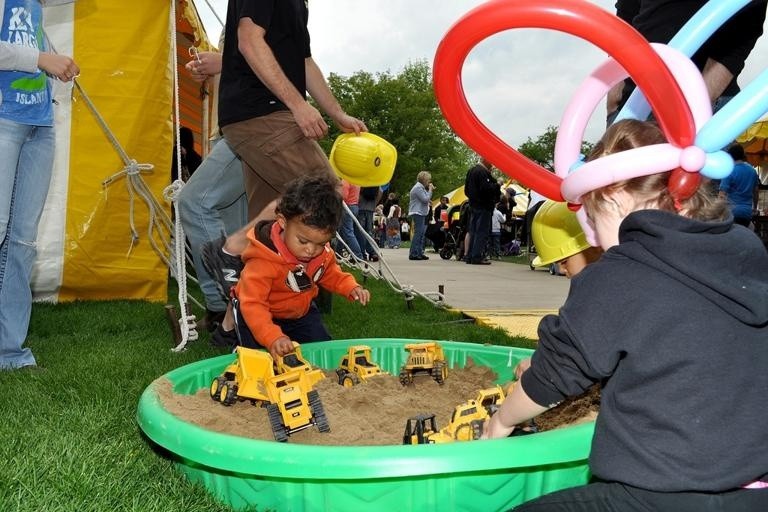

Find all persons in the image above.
[485,118,768,511]
[370,157,606,282]
[713,138,763,230]
[0,1,80,371]
[606,1,768,137]
[234,178,369,355]
[174,2,247,349]
[203,0,367,355]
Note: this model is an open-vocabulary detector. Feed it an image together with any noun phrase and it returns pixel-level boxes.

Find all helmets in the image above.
[328,132,398,187]
[532,199,592,268]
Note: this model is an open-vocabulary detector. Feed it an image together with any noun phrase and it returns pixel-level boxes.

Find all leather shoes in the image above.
[197,306,226,329]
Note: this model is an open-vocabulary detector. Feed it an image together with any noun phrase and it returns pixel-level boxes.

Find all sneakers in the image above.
[465,256,491,265]
[208,324,239,346]
[200,237,245,305]
[409,254,429,260]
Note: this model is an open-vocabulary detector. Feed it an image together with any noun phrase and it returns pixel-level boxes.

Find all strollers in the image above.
[439,200,487,262]
